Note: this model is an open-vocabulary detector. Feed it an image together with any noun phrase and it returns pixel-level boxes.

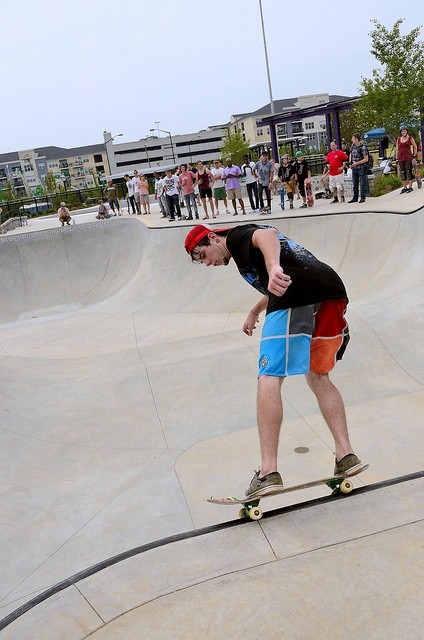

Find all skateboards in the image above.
[412,156,423,189]
[303,178,314,208]
[260,206,271,215]
[206,464,369,520]
[125,197,130,215]
[181,214,188,221]
[278,183,285,210]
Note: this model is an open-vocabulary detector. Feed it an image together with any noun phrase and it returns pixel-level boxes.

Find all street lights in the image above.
[6,165,15,198]
[105,134,123,175]
[189,136,202,165]
[150,128,176,164]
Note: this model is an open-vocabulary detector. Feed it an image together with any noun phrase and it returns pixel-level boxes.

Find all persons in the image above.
[196,160,218,220]
[162,168,180,221]
[270,159,281,192]
[57,202,71,226]
[188,162,201,206]
[294,150,312,208]
[154,173,169,218]
[137,174,151,214]
[253,151,273,215]
[210,159,230,215]
[341,143,350,173]
[102,179,122,216]
[395,126,418,194]
[132,170,142,213]
[320,154,331,195]
[241,155,260,210]
[182,222,364,496]
[348,133,369,203]
[178,164,199,220]
[96,200,112,219]
[174,166,183,207]
[124,174,138,214]
[326,142,348,204]
[278,154,295,209]
[219,158,246,216]
[153,172,162,212]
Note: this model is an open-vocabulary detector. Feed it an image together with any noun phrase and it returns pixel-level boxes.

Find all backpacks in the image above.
[359,143,374,171]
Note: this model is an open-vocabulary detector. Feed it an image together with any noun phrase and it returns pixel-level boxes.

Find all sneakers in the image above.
[331,454,364,475]
[360,199,366,202]
[349,199,356,202]
[400,188,405,193]
[340,199,344,202]
[407,189,413,192]
[330,200,338,203]
[244,473,284,497]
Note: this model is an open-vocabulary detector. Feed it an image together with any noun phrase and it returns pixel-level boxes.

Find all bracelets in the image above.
[251,310,259,317]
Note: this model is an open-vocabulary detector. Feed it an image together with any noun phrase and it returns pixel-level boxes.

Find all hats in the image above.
[295,151,304,159]
[281,155,290,160]
[59,202,67,207]
[184,224,232,252]
[399,125,408,131]
